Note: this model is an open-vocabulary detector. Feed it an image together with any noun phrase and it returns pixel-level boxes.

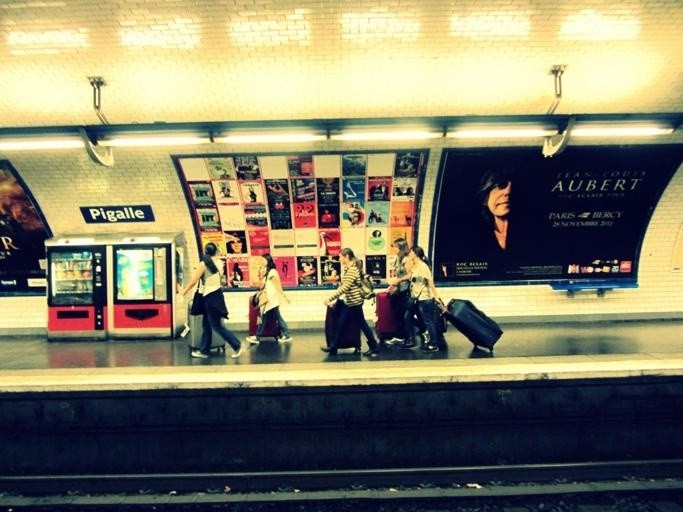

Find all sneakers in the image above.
[231,341,244,358]
[246,336,260,344]
[384,329,440,353]
[278,335,292,343]
[191,350,208,358]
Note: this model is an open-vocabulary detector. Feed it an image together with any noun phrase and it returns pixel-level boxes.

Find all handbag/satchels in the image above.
[389,289,419,313]
[191,293,204,315]
[258,291,268,307]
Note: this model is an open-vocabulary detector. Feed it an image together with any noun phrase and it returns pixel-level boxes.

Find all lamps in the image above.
[0,112,683,150]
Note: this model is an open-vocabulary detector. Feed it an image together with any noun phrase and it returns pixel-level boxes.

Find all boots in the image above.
[321,344,337,354]
[363,340,380,356]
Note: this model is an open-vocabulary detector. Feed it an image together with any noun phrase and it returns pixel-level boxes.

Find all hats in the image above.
[300,256,314,263]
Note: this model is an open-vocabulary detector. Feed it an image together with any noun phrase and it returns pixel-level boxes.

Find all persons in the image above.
[478,164,515,250]
[394,187,404,197]
[230,263,242,286]
[401,245,442,352]
[369,231,386,251]
[246,253,293,345]
[324,261,329,277]
[369,181,391,200]
[373,263,381,286]
[281,259,288,278]
[268,183,289,196]
[405,187,414,195]
[386,237,416,350]
[321,209,336,223]
[348,204,362,224]
[319,249,381,357]
[320,232,330,256]
[344,182,358,201]
[230,235,243,254]
[368,209,375,222]
[181,243,243,359]
[235,159,259,180]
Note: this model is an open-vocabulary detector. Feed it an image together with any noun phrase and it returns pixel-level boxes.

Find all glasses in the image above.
[487,179,508,191]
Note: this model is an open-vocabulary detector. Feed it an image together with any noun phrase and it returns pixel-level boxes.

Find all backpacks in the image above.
[343,264,376,300]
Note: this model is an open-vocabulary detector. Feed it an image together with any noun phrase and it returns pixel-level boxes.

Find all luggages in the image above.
[433,299,503,352]
[326,304,361,355]
[376,292,404,345]
[188,300,225,353]
[249,295,282,342]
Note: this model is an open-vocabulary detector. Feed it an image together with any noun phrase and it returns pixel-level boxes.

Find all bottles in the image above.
[233,271,238,287]
